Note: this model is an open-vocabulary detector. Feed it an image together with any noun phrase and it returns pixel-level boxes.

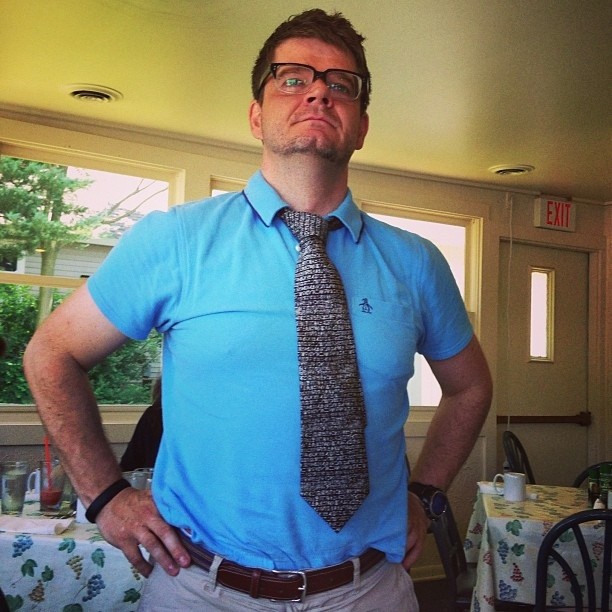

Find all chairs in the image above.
[534,509,612,612]
[570,461,612,489]
[429,504,478,611]
[501,428,537,486]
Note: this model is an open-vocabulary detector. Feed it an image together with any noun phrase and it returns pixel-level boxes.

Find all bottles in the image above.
[69,486,77,510]
[588,470,599,508]
[599,465,612,504]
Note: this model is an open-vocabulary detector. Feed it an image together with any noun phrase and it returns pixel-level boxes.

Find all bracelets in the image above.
[85,478,132,524]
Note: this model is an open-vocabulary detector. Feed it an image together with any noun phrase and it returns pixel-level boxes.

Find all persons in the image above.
[20,6,496,611]
[114,374,163,475]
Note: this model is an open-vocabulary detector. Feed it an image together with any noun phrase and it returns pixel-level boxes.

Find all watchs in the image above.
[406,481,448,522]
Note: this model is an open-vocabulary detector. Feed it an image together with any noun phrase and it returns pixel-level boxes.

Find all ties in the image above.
[279,204,373,536]
[165,537,389,606]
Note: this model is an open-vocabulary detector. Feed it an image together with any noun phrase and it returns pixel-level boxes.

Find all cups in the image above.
[608,490,612,510]
[135,468,153,490]
[1,461,27,517]
[28,468,41,494]
[40,460,66,511]
[122,471,146,489]
[493,472,526,502]
[77,498,91,524]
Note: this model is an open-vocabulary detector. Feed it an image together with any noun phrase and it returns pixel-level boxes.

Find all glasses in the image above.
[255,62,368,105]
